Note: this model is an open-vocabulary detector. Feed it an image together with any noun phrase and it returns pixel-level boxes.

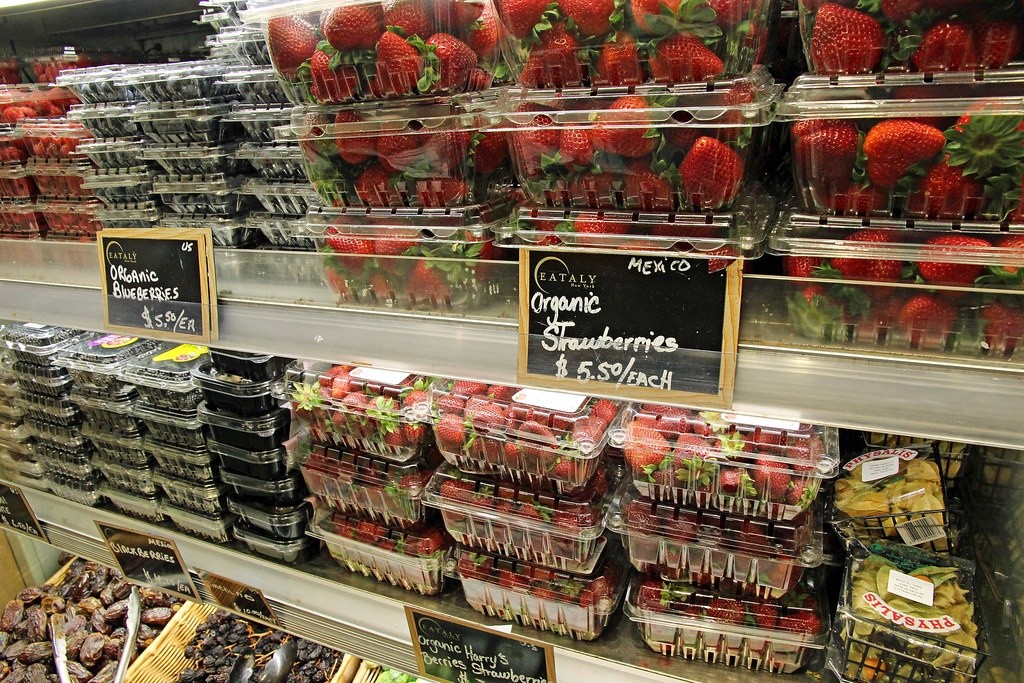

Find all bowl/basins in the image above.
[1,0,1024,683]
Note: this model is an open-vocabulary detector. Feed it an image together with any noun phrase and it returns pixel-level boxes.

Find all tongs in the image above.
[50,586,141,683]
[225,641,297,683]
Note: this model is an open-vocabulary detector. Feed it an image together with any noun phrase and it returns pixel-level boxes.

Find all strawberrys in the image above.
[612,395,826,675]
[781,0,1024,366]
[262,0,779,274]
[290,362,625,642]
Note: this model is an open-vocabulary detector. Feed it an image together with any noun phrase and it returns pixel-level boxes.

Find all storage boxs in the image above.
[0,0,1024,683]
